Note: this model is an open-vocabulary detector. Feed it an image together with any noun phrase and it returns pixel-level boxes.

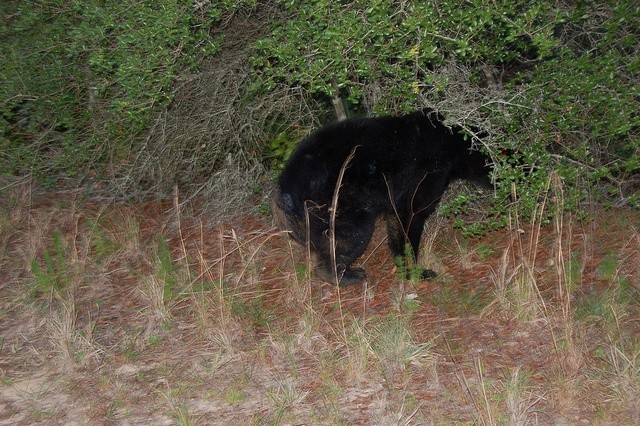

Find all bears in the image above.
[272,106,538,288]
